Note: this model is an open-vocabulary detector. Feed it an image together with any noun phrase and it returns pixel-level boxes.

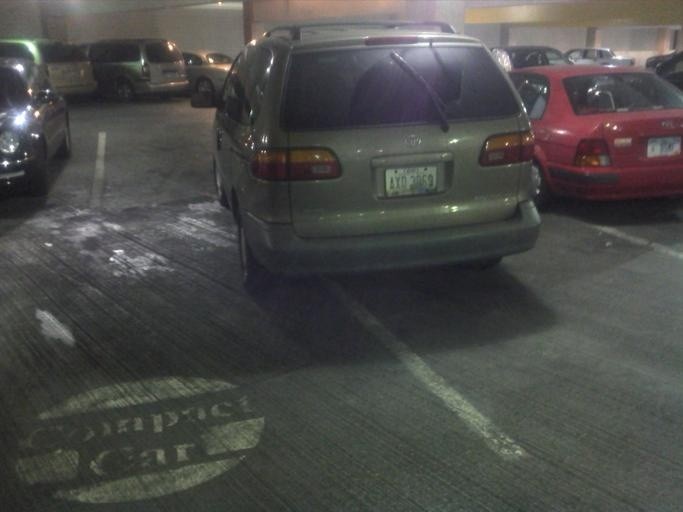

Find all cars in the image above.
[214,21,682,291]
[0,38,238,196]
